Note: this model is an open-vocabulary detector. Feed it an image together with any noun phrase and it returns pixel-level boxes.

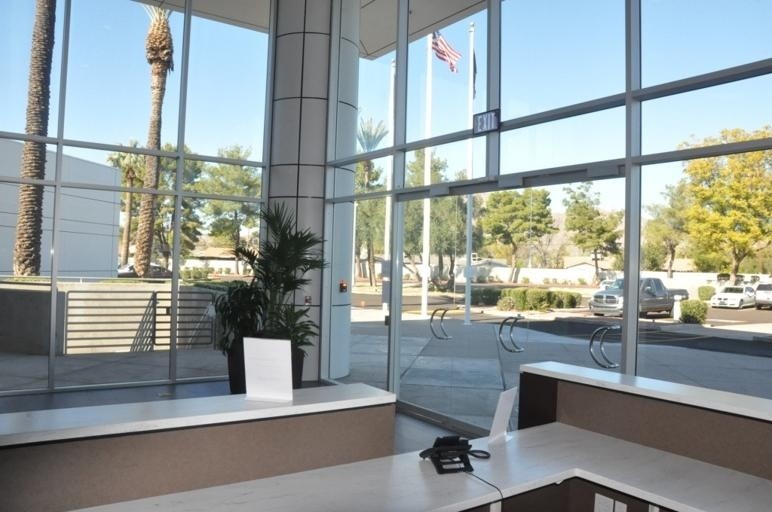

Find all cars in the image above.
[710,285,756,308]
[599,279,615,290]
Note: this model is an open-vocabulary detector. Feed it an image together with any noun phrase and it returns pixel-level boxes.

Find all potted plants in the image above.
[214,200,331,394]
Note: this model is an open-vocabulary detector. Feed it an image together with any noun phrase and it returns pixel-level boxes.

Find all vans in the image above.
[753,283,772,309]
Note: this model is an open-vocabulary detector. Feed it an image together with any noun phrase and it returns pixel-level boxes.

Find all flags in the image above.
[430,30,464,74]
[473,50,478,98]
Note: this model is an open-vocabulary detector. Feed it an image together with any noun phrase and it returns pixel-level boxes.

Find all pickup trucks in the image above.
[589,276,689,317]
